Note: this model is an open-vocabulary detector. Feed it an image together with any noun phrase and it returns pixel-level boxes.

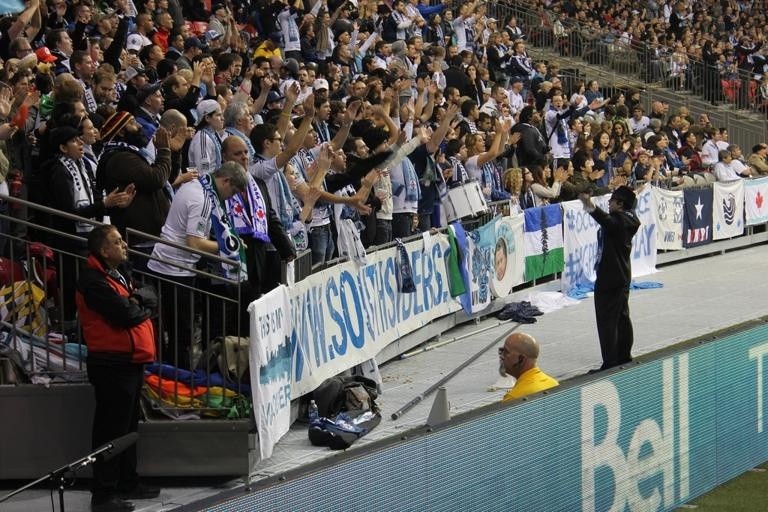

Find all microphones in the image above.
[110,431,140,455]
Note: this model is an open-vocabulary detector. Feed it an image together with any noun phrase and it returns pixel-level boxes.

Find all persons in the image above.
[497,331,560,405]
[1,1,768,373]
[75,223,161,512]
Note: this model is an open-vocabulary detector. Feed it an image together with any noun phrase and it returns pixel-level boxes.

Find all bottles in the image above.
[308,399,319,426]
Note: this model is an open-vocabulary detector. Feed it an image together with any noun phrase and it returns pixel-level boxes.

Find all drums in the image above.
[440,177,489,225]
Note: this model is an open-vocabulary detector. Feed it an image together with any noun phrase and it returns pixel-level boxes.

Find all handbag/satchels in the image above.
[314,375,377,416]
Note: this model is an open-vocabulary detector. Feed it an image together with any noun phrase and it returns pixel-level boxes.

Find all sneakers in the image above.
[91,479,160,512]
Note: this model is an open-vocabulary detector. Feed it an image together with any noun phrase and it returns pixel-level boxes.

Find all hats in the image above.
[630,87,646,113]
[34,47,58,64]
[97,106,135,144]
[266,91,286,104]
[486,18,500,25]
[205,30,224,43]
[94,8,160,101]
[194,99,220,127]
[510,76,526,84]
[333,30,345,43]
[184,37,208,49]
[612,185,636,207]
[313,79,329,91]
[212,4,225,12]
[268,32,284,48]
[279,58,299,73]
